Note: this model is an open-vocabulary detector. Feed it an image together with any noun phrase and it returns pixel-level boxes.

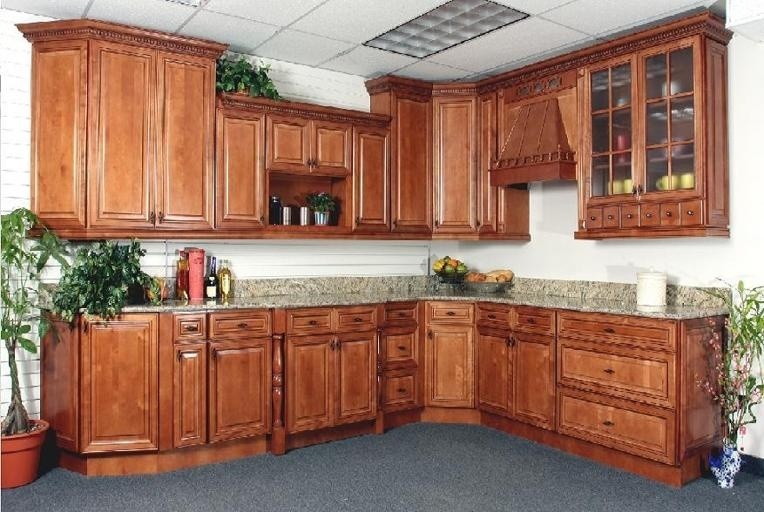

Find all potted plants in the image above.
[1,207,74,489]
[216,53,284,103]
[306,192,336,226]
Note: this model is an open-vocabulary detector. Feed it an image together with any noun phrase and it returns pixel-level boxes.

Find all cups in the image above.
[604,173,694,195]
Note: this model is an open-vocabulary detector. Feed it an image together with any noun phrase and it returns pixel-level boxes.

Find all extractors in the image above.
[486,98,577,187]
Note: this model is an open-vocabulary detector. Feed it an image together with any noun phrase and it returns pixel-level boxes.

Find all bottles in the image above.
[175,250,189,300]
[205,256,219,300]
[269,195,309,225]
[635,266,666,306]
[614,128,627,163]
[218,259,233,298]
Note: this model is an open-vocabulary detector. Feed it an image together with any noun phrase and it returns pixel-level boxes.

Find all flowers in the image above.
[695,276,764,447]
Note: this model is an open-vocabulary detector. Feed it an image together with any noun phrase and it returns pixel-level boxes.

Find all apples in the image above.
[456,263,468,273]
[444,256,451,264]
[446,265,455,274]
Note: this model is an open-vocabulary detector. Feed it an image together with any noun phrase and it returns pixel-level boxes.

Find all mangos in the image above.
[433,259,445,272]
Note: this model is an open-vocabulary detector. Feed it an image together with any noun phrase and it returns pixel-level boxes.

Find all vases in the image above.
[710,438,742,489]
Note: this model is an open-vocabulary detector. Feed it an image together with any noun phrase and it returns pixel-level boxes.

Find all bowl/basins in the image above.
[461,281,511,293]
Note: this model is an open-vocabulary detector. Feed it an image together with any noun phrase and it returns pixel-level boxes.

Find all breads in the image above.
[465,269,514,282]
